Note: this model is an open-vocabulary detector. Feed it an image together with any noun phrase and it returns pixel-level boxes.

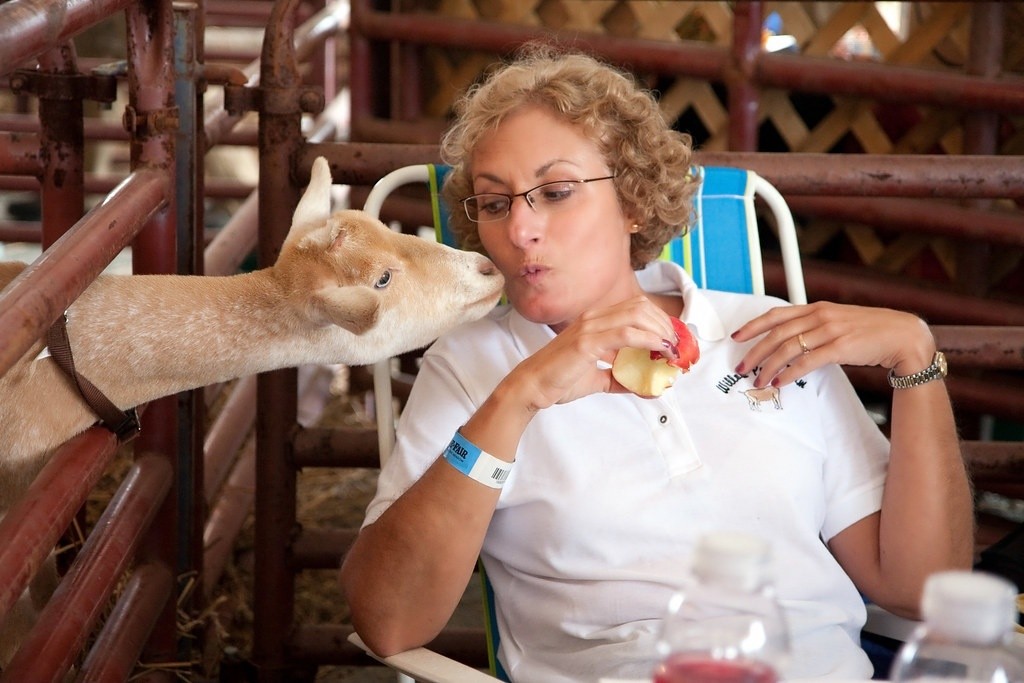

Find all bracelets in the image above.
[444,426,516,489]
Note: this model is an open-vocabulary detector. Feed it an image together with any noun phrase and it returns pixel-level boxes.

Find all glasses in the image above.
[459,176,618,223]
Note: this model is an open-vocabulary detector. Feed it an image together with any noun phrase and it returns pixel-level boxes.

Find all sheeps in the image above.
[1,157,505,468]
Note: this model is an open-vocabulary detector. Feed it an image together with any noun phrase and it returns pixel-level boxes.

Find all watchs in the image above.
[887,351,951,389]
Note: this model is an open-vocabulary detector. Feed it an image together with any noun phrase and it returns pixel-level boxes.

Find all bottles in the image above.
[888,570,1024,683]
[650,529,792,683]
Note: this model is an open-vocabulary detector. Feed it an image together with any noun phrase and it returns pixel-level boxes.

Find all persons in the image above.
[336,44,973,683]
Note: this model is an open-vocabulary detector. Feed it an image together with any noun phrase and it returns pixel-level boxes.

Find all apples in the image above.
[612,316,700,399]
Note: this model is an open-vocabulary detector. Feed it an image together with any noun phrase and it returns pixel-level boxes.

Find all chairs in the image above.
[348,163,813,682]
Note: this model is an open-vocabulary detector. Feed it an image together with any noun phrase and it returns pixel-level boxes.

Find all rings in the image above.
[798,333,811,354]
